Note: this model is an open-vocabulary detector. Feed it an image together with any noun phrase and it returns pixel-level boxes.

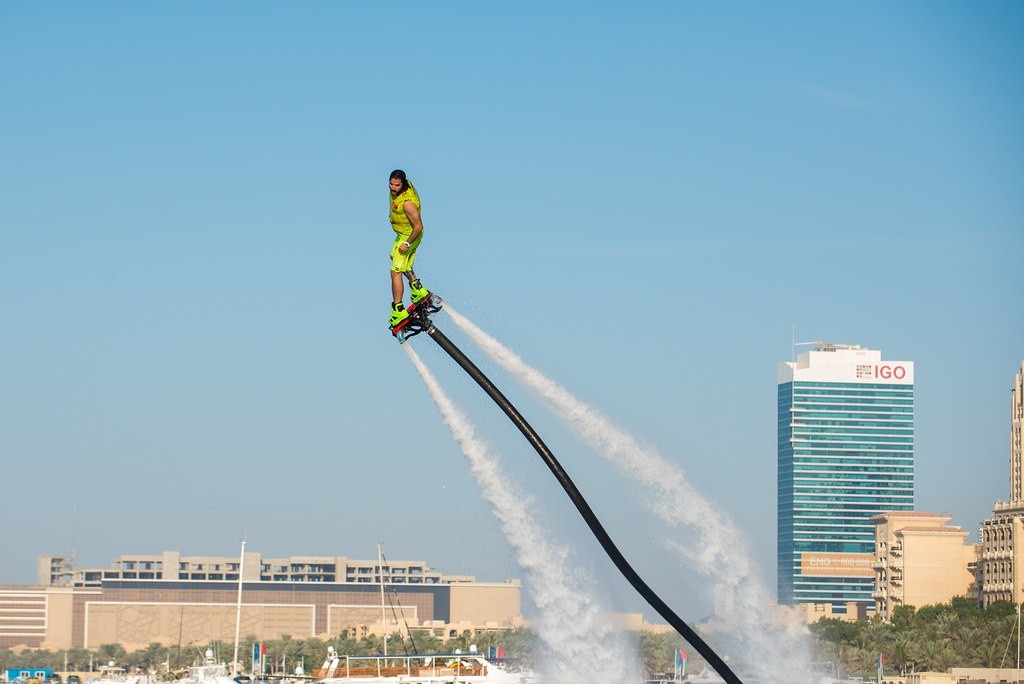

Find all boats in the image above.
[310,645,523,684]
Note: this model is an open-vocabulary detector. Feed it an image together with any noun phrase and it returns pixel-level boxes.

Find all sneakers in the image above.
[388,300,409,327]
[409,278,430,304]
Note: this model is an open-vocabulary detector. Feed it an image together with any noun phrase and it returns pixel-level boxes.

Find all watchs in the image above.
[404,241,412,247]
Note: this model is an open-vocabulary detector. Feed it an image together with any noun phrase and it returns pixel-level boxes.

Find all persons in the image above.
[389,169,431,328]
